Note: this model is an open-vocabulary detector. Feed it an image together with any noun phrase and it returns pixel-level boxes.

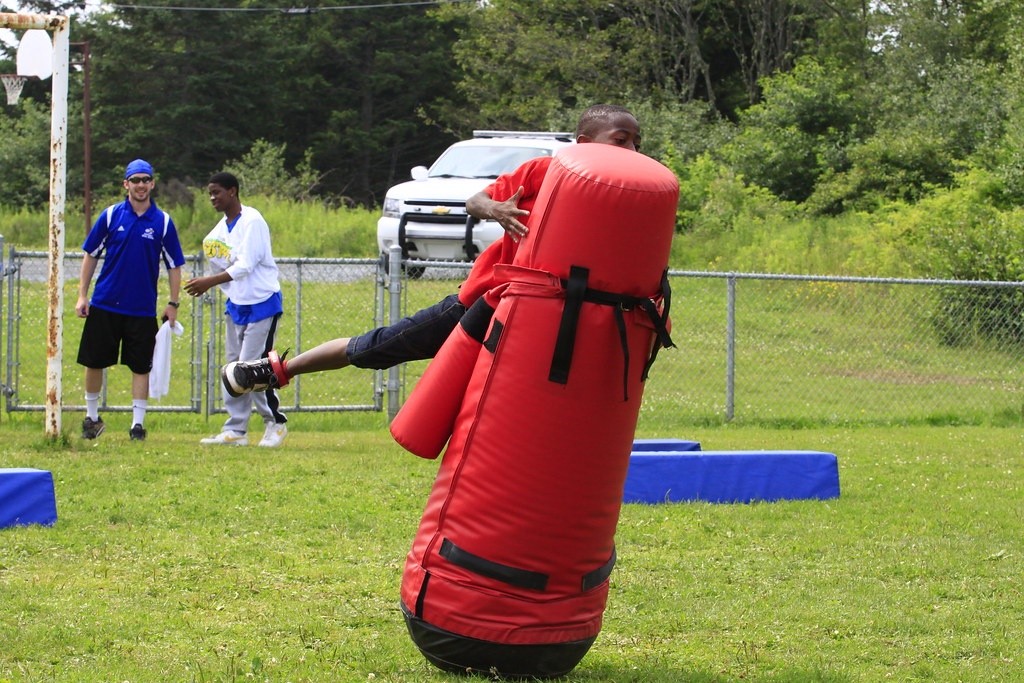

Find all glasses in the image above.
[127,177,152,184]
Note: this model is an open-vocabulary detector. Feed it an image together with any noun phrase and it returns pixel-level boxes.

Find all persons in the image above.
[75,160,184,441]
[223,104,643,398]
[184,171,288,448]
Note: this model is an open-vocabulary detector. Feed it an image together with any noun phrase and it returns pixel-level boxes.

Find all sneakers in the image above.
[199,425,248,447]
[221,349,290,397]
[128,423,146,439]
[81,415,105,440]
[259,422,288,447]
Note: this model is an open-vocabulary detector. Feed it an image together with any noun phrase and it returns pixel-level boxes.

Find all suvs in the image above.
[376,130,579,280]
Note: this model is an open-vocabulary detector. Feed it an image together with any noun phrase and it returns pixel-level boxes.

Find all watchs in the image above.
[168,301,179,308]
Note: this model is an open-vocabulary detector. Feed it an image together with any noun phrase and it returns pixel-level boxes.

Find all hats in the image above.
[127,158,154,180]
